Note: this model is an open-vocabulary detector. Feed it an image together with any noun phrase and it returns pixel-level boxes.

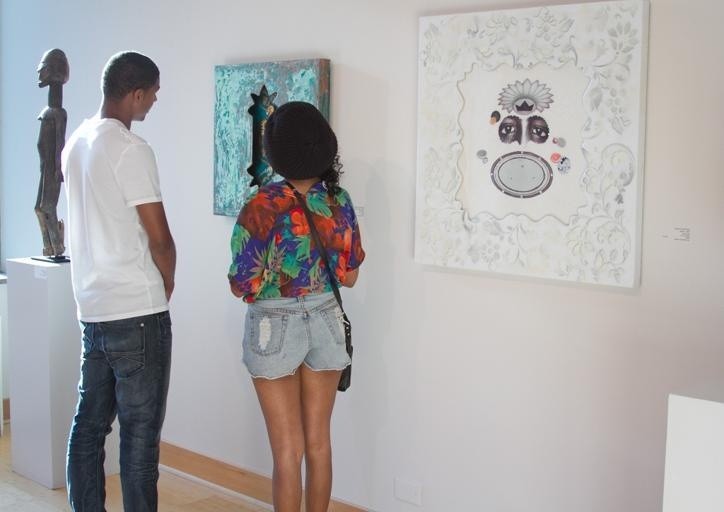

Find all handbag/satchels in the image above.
[337,322,353,392]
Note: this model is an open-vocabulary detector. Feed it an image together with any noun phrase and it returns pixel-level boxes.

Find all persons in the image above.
[228,101,365,512]
[61,51,176,512]
[34,48,69,256]
